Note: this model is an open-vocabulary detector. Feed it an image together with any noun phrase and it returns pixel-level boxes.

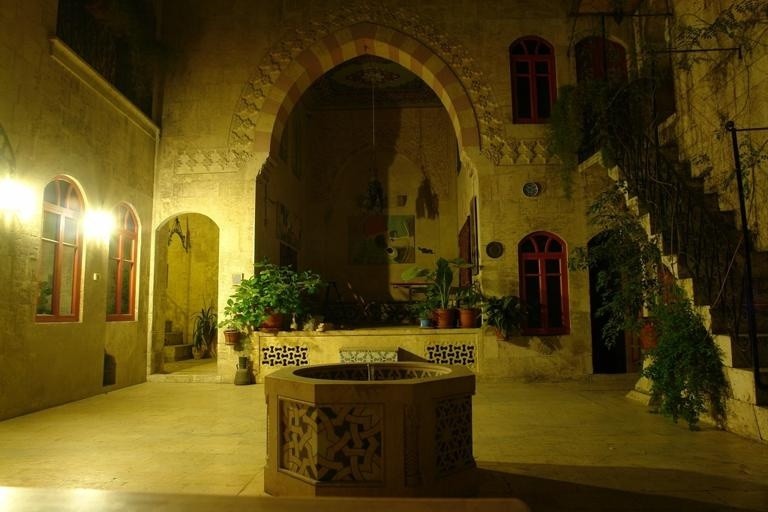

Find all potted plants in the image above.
[400,247,522,341]
[191,306,217,359]
[219,256,328,345]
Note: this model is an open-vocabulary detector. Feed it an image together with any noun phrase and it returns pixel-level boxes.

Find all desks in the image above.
[391,276,436,305]
